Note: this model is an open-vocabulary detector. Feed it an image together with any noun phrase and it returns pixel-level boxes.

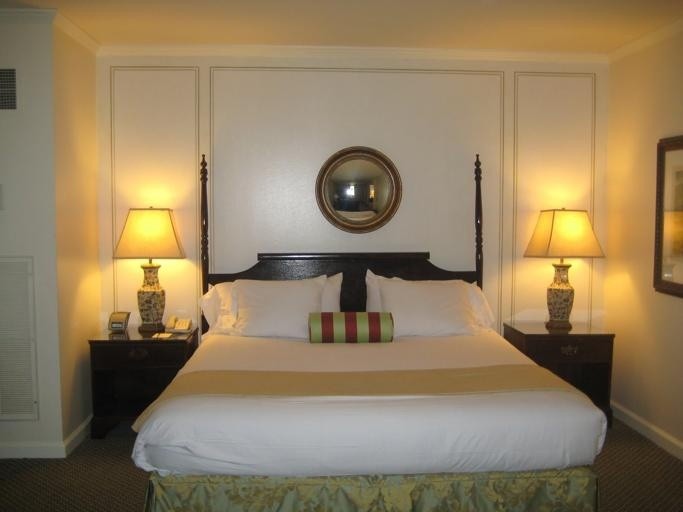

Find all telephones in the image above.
[165,315,193,333]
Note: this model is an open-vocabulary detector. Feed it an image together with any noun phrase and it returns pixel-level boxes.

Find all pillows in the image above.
[198,269,494,344]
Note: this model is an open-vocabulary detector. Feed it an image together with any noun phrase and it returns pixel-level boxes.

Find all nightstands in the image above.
[86,323,198,438]
[501,321,615,427]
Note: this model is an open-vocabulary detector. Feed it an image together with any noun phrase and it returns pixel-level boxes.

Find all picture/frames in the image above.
[652,134,681,300]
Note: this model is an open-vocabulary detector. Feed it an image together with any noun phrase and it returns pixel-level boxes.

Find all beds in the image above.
[131,252,607,510]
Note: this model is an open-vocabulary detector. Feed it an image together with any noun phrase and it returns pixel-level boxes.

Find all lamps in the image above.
[111,206,186,333]
[522,208,606,329]
